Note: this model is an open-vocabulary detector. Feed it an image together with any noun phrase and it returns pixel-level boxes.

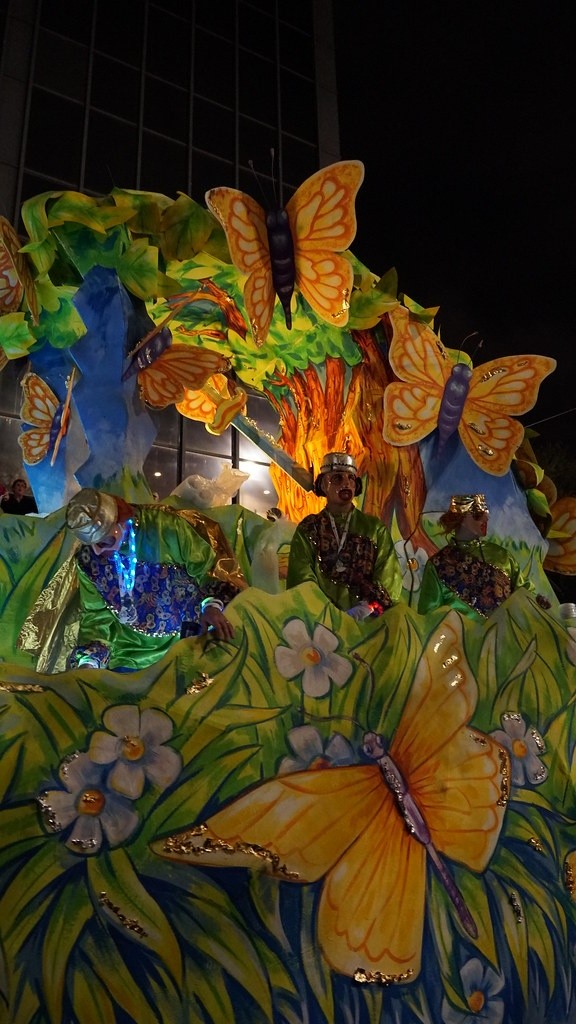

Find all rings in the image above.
[225,621,229,623]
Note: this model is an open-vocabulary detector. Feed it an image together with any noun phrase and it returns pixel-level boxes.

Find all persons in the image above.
[63,487,242,670]
[285,453,404,623]
[0,479,38,515]
[417,495,552,621]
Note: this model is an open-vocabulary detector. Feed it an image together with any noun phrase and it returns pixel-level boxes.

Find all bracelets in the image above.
[201,596,223,613]
[78,656,98,668]
